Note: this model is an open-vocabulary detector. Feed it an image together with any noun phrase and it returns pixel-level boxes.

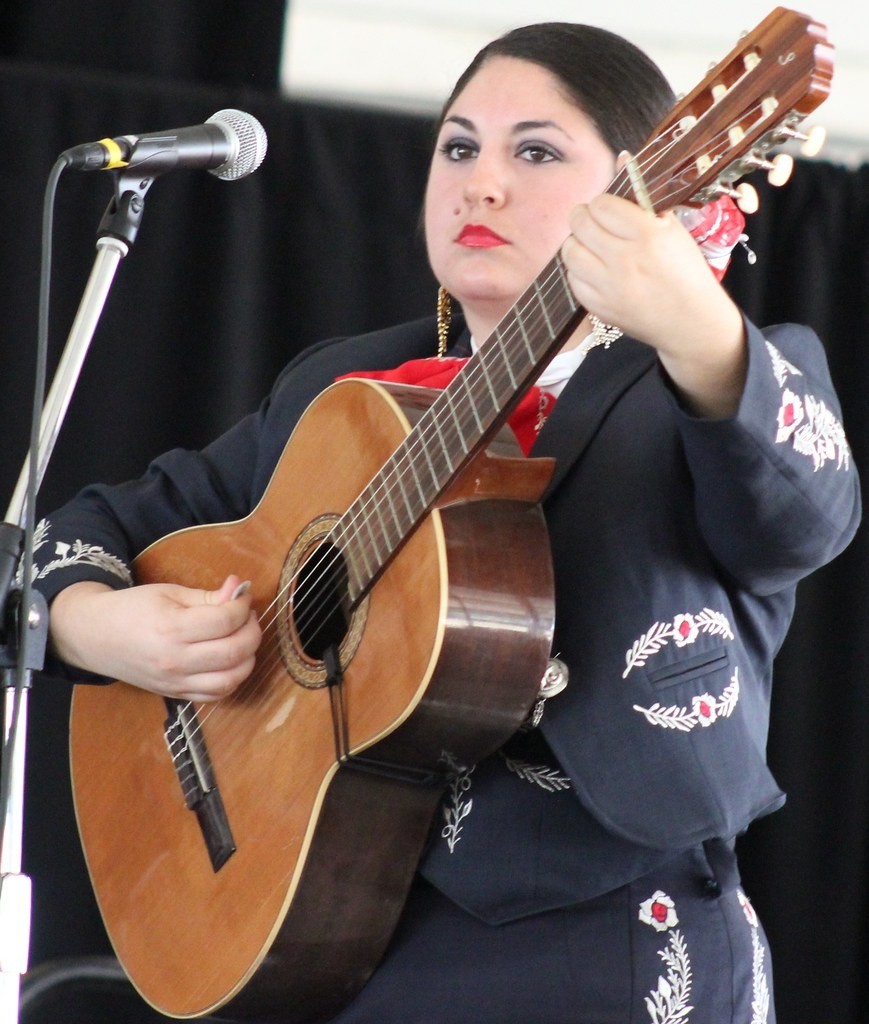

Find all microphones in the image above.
[56,108,267,182]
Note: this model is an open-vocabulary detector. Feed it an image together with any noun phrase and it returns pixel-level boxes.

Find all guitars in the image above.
[65,5,837,1023]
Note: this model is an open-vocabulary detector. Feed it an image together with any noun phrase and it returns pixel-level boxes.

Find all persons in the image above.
[12,24,861,1024]
